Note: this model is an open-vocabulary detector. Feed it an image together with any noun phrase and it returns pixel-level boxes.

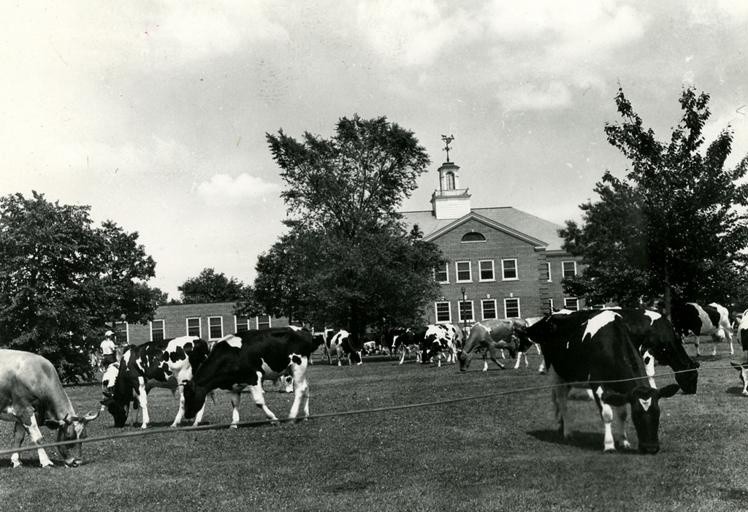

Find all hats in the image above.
[105,331,115,336]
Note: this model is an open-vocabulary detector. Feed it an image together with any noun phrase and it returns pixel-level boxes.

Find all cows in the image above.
[0,347,98,469]
[99,302,748,454]
